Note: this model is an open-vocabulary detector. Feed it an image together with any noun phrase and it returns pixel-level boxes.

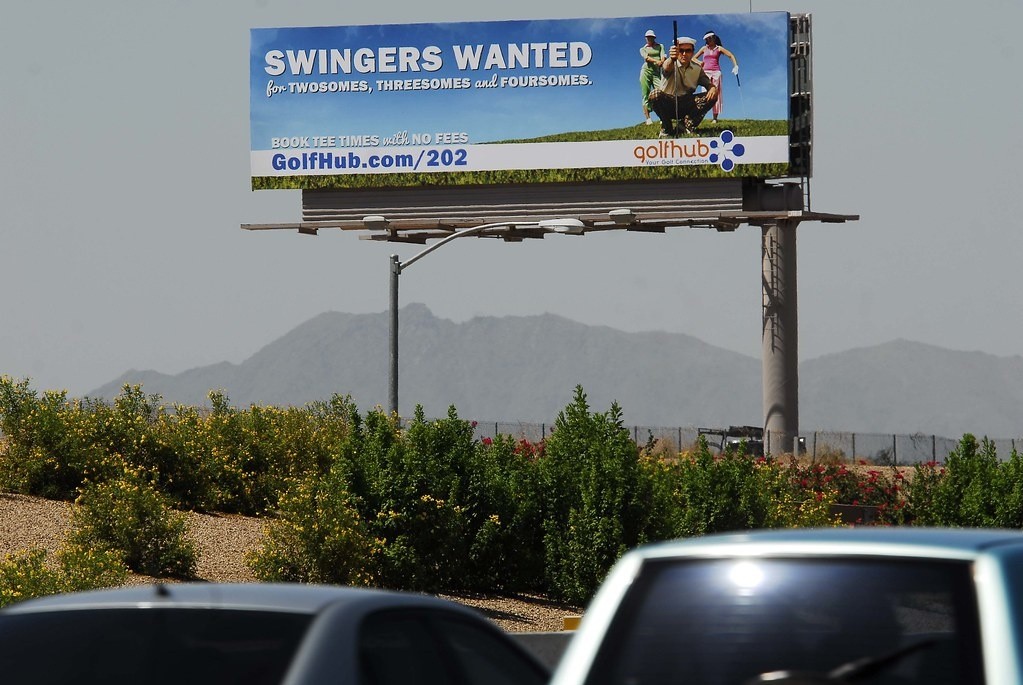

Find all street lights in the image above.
[389,217,587,421]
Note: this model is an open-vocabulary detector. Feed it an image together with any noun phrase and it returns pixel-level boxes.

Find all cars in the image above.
[543,525,1023,684]
[0,581,558,684]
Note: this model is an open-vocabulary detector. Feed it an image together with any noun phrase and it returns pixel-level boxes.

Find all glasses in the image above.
[679,49,691,52]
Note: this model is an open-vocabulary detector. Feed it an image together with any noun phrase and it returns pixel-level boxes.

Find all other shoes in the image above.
[659,121,674,138]
[646,118,654,125]
[711,119,718,124]
[676,120,693,134]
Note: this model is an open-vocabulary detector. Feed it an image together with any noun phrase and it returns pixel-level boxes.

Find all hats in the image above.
[645,30,656,38]
[703,33,714,39]
[672,37,697,45]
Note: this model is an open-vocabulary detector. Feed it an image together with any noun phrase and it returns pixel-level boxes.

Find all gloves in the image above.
[732,66,739,75]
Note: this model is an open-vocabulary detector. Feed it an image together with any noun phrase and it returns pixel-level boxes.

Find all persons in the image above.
[650,36,718,137]
[692,31,738,123]
[640,30,666,126]
